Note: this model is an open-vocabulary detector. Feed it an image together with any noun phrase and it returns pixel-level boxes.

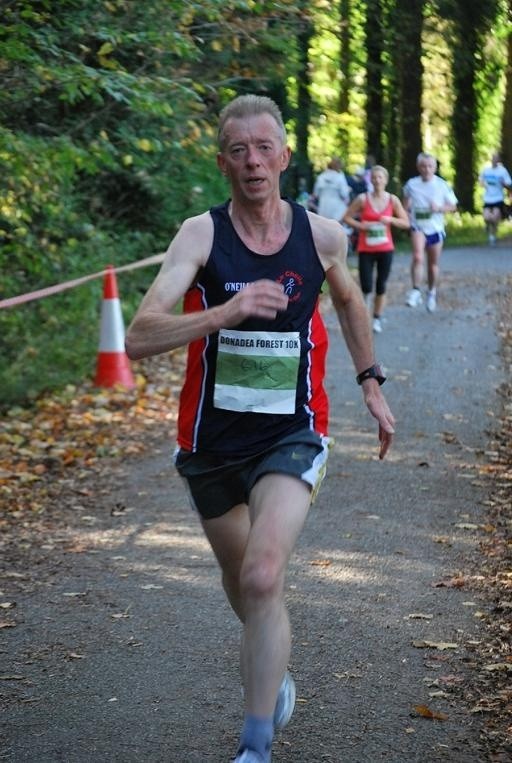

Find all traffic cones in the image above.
[91,267,139,393]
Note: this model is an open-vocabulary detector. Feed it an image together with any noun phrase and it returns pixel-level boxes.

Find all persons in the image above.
[296,153,458,333]
[126,96,394,762]
[478,155,512,246]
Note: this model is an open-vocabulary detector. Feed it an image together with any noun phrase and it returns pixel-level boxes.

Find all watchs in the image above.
[355,363,386,385]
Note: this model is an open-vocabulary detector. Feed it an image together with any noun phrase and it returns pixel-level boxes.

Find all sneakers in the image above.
[231,669,296,763]
[407,289,437,312]
[373,318,382,332]
